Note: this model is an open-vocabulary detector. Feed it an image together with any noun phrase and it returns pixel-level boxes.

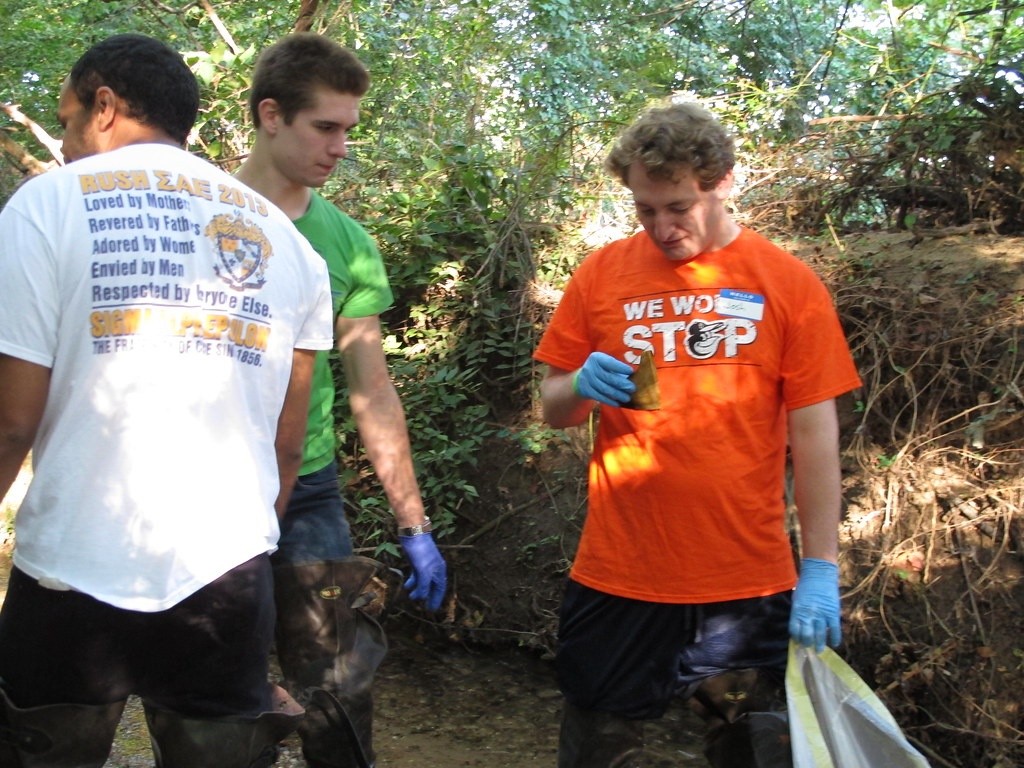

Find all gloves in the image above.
[787,558,842,654]
[397,534,448,611]
[575,352,637,409]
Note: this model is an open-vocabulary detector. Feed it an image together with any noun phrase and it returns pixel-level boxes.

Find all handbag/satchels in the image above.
[783,635,932,768]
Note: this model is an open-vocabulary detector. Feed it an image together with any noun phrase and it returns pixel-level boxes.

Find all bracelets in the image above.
[398,516,433,537]
[573,369,582,397]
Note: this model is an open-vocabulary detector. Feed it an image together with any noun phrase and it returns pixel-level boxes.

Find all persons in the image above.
[0,34,335,768]
[232,34,446,767]
[532,104,862,768]
